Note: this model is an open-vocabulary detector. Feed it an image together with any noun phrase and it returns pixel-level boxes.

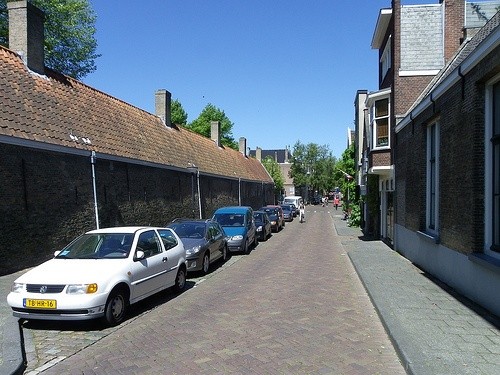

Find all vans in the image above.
[282,196,304,214]
[211,206,258,255]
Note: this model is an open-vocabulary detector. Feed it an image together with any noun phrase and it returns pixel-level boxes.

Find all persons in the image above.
[298,201,306,221]
[322,195,329,207]
[334,197,340,210]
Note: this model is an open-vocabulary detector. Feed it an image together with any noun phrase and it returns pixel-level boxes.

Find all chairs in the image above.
[105,239,122,249]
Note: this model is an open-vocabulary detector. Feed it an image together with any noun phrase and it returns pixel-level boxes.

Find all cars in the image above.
[153,218,229,276]
[277,205,296,222]
[283,204,298,218]
[7,226,188,327]
[252,211,272,241]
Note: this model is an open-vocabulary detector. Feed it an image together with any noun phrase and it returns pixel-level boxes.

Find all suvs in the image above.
[258,206,283,233]
